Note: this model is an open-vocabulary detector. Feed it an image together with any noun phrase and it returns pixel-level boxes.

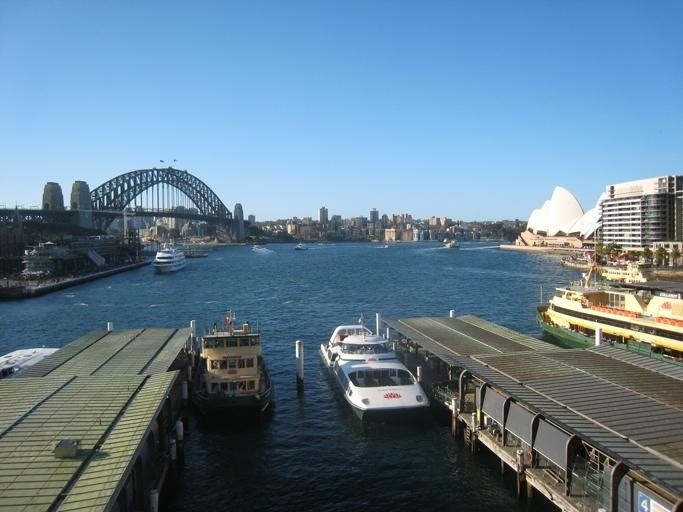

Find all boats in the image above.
[295,242,308,250]
[0,346,61,377]
[142,243,209,259]
[383,245,389,248]
[251,245,268,253]
[149,238,185,274]
[599,264,656,284]
[538,267,682,365]
[187,309,274,416]
[319,312,431,423]
[559,256,596,271]
[444,239,460,248]
